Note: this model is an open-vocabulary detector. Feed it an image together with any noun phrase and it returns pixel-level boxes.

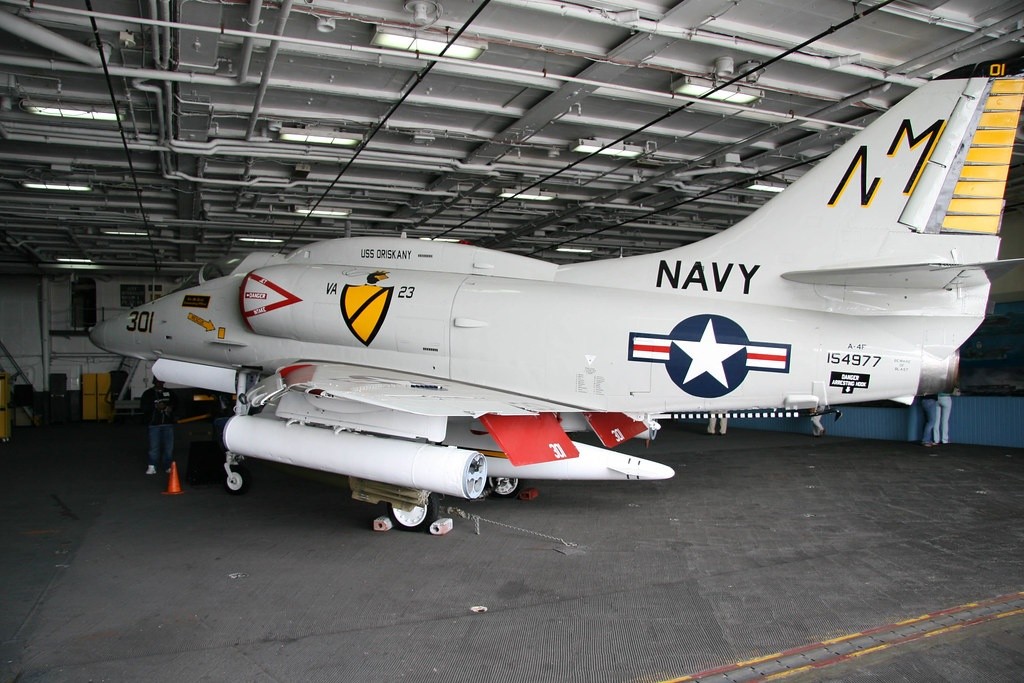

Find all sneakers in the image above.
[145,464,157,475]
[166,467,171,474]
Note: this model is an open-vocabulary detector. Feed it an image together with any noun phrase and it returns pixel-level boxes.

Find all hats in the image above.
[152,376,166,384]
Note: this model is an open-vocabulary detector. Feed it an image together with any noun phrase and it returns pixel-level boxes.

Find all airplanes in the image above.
[88,48,1024,536]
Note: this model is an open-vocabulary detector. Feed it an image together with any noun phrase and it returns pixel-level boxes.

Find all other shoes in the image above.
[929,440,938,446]
[718,433,725,435]
[924,443,932,447]
[704,433,716,435]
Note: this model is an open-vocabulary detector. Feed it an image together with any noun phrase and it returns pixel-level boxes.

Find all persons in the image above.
[708,417,728,435]
[811,414,826,435]
[904,383,952,448]
[140,376,181,474]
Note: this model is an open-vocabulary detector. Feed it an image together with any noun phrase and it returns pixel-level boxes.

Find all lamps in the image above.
[551,246,594,254]
[277,124,364,149]
[570,137,643,160]
[20,179,93,192]
[671,76,765,108]
[21,100,127,122]
[54,255,95,264]
[741,179,789,193]
[293,205,352,217]
[494,185,557,201]
[235,235,286,244]
[369,25,488,63]
[100,226,152,236]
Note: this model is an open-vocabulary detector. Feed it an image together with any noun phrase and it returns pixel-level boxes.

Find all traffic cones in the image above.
[160,460,187,495]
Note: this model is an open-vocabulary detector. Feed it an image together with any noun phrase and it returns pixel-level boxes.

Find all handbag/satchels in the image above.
[950,386,961,397]
